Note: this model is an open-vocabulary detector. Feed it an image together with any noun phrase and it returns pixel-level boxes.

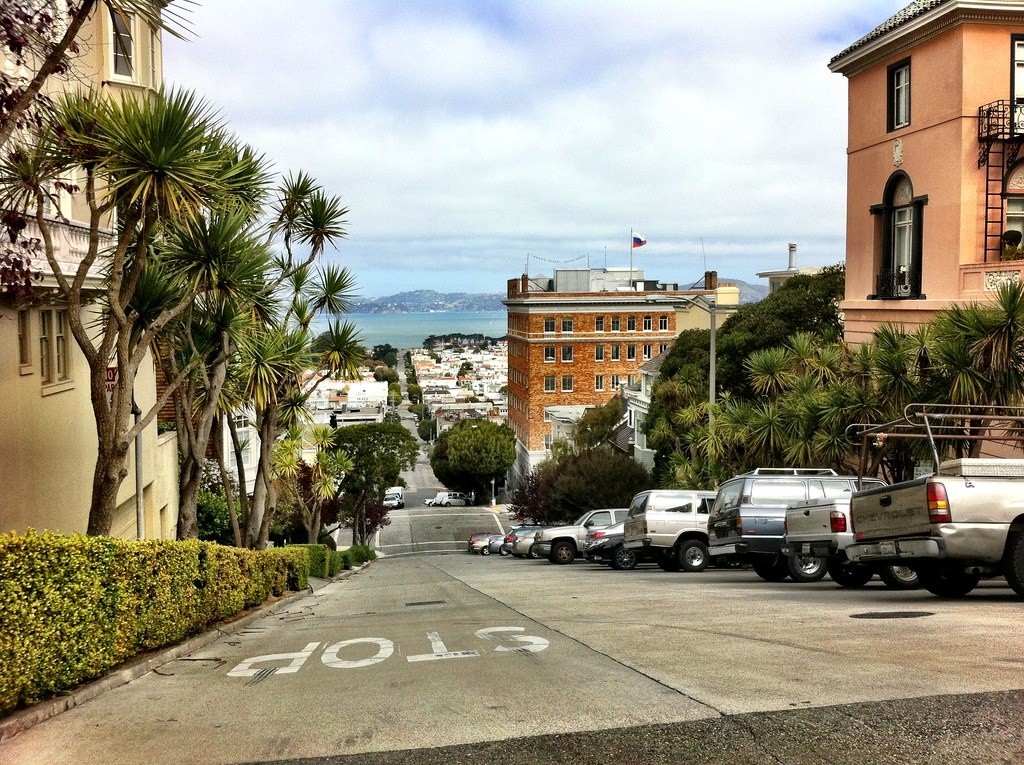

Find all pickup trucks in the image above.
[780,498,924,589]
[842,399,1022,601]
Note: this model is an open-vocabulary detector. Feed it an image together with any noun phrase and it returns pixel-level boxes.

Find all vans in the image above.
[424,491,472,507]
[382,487,406,509]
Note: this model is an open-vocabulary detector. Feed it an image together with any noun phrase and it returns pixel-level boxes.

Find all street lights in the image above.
[645,293,722,490]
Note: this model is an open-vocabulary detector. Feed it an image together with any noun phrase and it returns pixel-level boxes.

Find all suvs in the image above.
[708,463,895,582]
[624,488,759,573]
[534,507,632,564]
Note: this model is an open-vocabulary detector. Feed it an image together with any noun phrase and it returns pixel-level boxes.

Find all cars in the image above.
[583,534,685,572]
[469,524,603,562]
[586,519,631,550]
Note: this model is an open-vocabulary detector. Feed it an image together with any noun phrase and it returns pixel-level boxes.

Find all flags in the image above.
[632,231,647,248]
